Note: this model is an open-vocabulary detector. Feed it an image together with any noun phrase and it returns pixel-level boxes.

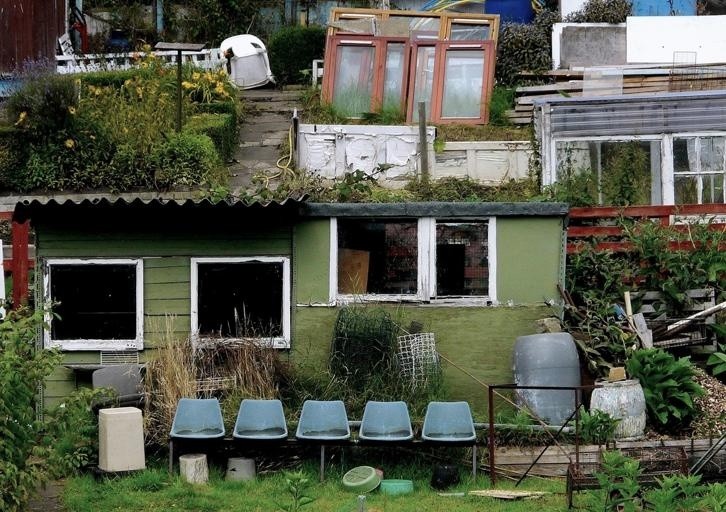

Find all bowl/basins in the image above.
[342,465,414,497]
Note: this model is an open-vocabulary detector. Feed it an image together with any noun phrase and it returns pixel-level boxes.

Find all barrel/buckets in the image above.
[512,333,582,426]
[179,453,208,485]
[224,455,255,482]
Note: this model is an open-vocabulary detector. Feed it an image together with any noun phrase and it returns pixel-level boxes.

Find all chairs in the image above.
[294,399,353,482]
[354,399,420,450]
[420,400,477,477]
[228,398,294,445]
[91,364,150,410]
[169,398,228,473]
[219,34,276,89]
